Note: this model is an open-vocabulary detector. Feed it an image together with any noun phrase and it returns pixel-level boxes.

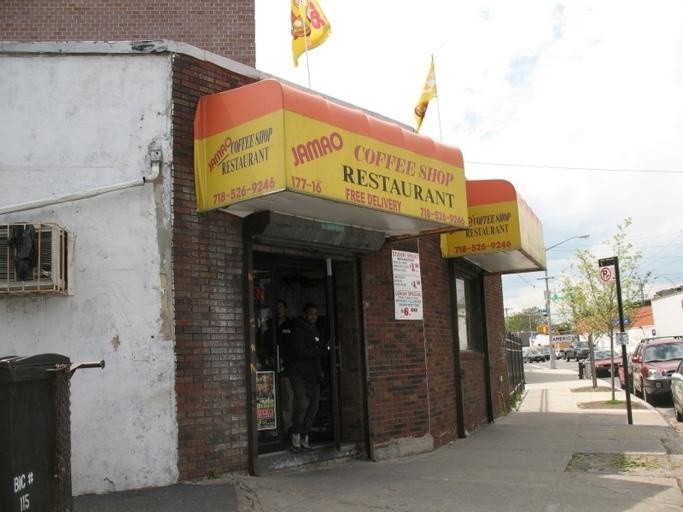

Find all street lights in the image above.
[539,233,593,369]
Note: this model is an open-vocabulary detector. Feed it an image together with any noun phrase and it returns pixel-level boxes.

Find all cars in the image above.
[522,335,683,421]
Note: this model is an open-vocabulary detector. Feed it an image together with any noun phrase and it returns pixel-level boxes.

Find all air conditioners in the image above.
[0,221,67,297]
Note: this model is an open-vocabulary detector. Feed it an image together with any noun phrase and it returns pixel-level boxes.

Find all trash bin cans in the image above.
[585,362,592,379]
[1,353,71,507]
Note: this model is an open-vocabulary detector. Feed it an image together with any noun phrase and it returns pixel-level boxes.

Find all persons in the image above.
[263,298,326,454]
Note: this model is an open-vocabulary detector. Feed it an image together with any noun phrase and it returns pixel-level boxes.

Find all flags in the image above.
[290,0,332,67]
[414,61,437,131]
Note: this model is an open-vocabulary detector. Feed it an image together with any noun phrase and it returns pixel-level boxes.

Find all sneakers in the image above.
[289,441,314,454]
